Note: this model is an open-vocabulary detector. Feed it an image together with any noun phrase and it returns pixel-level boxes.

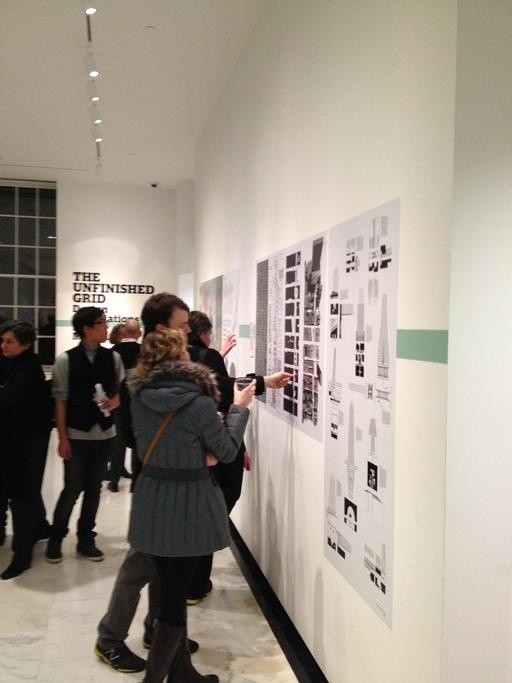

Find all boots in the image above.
[76,530,104,562]
[142,619,218,683]
[44,524,70,564]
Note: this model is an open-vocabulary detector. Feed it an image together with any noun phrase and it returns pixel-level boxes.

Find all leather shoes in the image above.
[36,519,50,541]
[106,481,119,492]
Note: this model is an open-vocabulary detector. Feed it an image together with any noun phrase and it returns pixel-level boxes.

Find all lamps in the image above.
[84,0,98,43]
[87,55,103,159]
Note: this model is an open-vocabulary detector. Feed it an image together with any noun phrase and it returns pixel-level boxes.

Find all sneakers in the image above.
[94,640,146,673]
[186,579,213,605]
[1,553,32,580]
[143,622,199,654]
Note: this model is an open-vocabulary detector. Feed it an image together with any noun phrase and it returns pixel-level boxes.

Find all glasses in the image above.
[92,320,107,325]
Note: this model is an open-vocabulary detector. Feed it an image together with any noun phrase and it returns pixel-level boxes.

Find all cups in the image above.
[235,377,253,392]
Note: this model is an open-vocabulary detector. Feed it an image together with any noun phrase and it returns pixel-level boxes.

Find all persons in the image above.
[188,308,236,359]
[1,317,53,580]
[127,327,257,683]
[92,290,295,674]
[108,319,146,492]
[45,305,126,564]
[1,314,71,542]
[185,328,252,604]
